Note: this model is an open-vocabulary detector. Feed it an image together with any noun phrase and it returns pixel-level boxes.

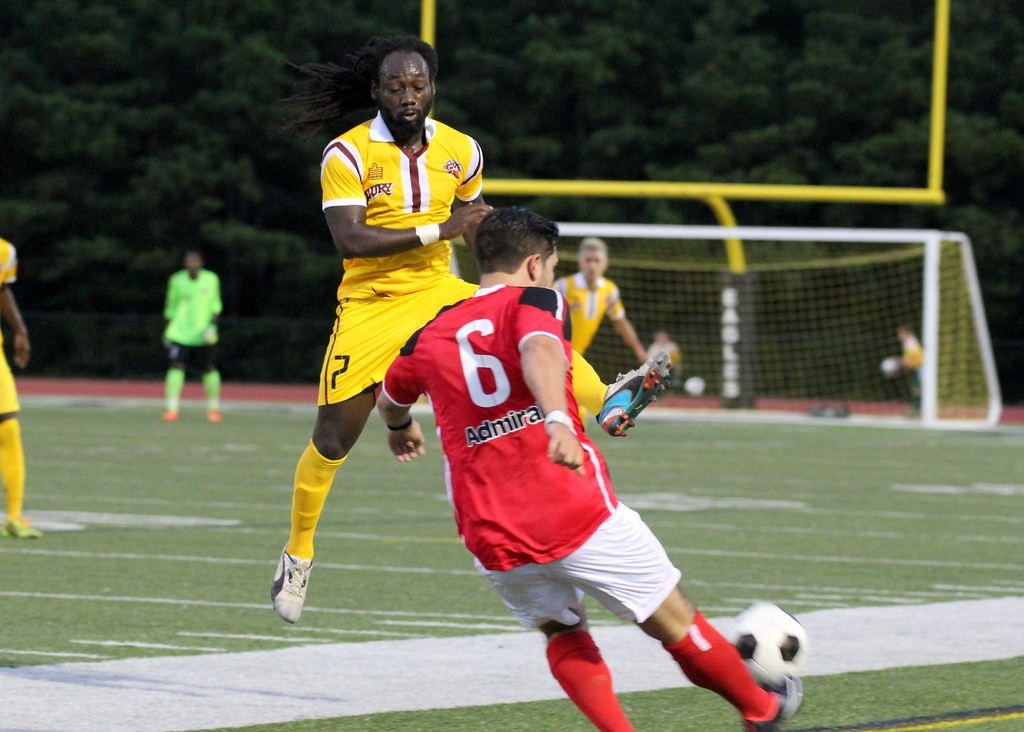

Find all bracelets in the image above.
[415,223,440,246]
[544,411,577,437]
[387,416,412,431]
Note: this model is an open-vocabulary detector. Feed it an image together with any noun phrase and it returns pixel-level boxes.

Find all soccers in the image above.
[881,357,900,375]
[683,376,706,397]
[724,603,808,693]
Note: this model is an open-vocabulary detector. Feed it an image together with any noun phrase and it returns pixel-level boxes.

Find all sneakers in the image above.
[745,676,803,732]
[271,541,313,625]
[0,517,40,538]
[595,350,672,438]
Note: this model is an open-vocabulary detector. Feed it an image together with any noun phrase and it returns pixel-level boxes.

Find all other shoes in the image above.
[161,410,179,422]
[208,411,220,423]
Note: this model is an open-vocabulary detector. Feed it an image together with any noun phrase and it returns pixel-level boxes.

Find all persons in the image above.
[553,238,682,430]
[0,237,45,540]
[272,36,673,624]
[895,325,924,418]
[161,250,224,424]
[377,207,804,732]
